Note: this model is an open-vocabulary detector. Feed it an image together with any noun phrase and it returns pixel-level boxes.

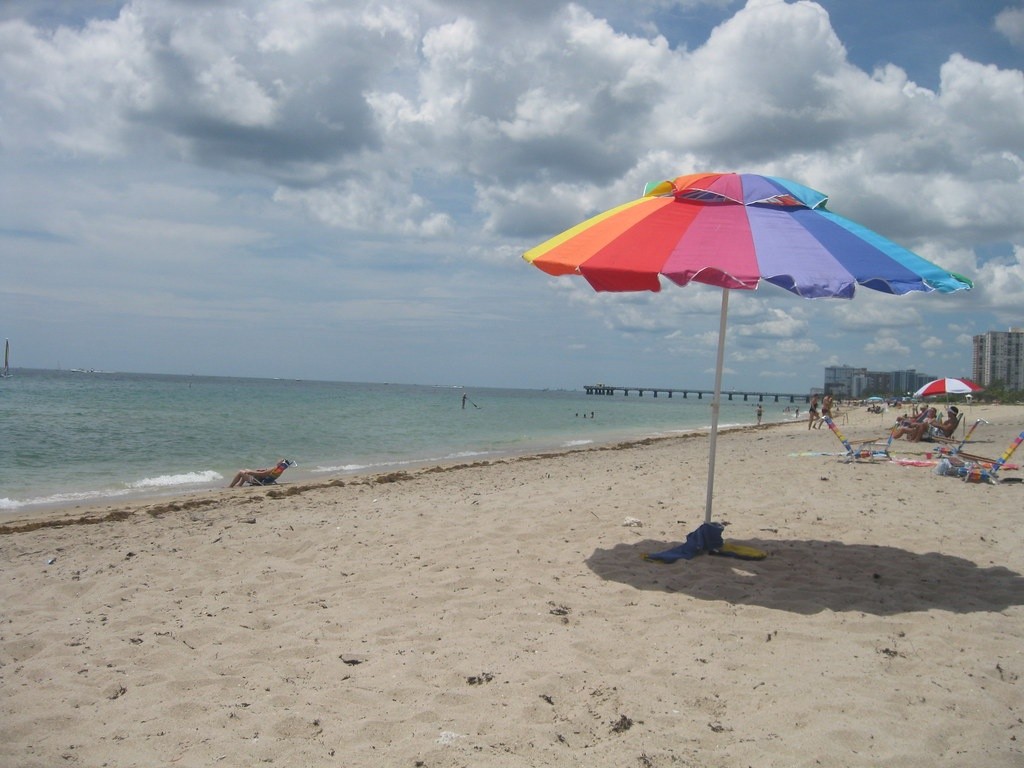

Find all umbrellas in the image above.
[522,173,974,526]
[869,397,882,400]
[913,377,981,408]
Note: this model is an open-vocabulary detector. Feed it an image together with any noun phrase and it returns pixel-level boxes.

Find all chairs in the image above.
[243,461,297,486]
[813,408,1024,487]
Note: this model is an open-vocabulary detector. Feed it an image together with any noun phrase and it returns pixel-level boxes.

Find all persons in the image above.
[895,405,959,442]
[809,394,820,430]
[818,392,833,429]
[783,406,790,412]
[873,404,881,410]
[755,405,764,425]
[795,406,800,418]
